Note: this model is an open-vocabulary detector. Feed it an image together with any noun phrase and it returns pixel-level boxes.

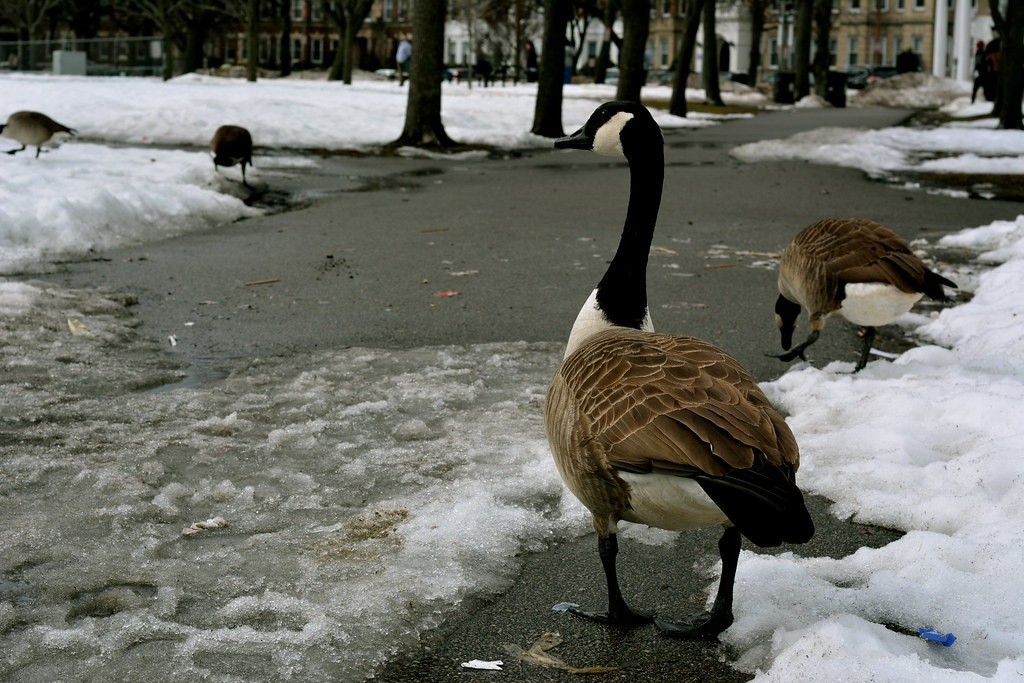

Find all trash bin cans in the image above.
[819,69,847,107]
[771,69,794,103]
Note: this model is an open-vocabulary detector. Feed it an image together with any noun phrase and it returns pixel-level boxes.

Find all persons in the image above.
[396,37,412,86]
[970,39,985,103]
[525,41,540,82]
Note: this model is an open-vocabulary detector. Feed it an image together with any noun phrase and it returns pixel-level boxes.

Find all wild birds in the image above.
[546,98,816,643]
[0,111,77,158]
[209,125,253,185]
[763,218,958,374]
